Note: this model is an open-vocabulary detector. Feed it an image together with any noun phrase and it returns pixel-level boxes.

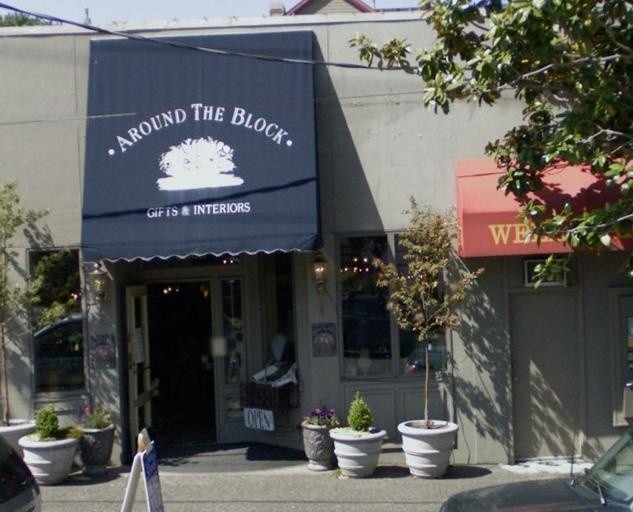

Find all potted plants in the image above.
[368,191,486,479]
[326,389,388,478]
[16,403,79,487]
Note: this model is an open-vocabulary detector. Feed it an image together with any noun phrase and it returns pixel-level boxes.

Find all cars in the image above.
[32,311,84,390]
[438,418,631,511]
[405,332,446,377]
[339,314,408,379]
[0,433,41,511]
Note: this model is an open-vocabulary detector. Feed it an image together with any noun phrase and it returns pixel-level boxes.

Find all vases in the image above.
[300,417,337,474]
[78,424,115,478]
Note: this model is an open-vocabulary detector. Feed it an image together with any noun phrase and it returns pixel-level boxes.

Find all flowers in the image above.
[298,400,339,428]
[79,402,115,429]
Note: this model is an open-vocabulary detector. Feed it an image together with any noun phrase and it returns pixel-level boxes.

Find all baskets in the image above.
[239,382,289,414]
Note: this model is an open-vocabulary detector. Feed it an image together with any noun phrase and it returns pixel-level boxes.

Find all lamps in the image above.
[307,247,328,295]
[87,260,110,303]
